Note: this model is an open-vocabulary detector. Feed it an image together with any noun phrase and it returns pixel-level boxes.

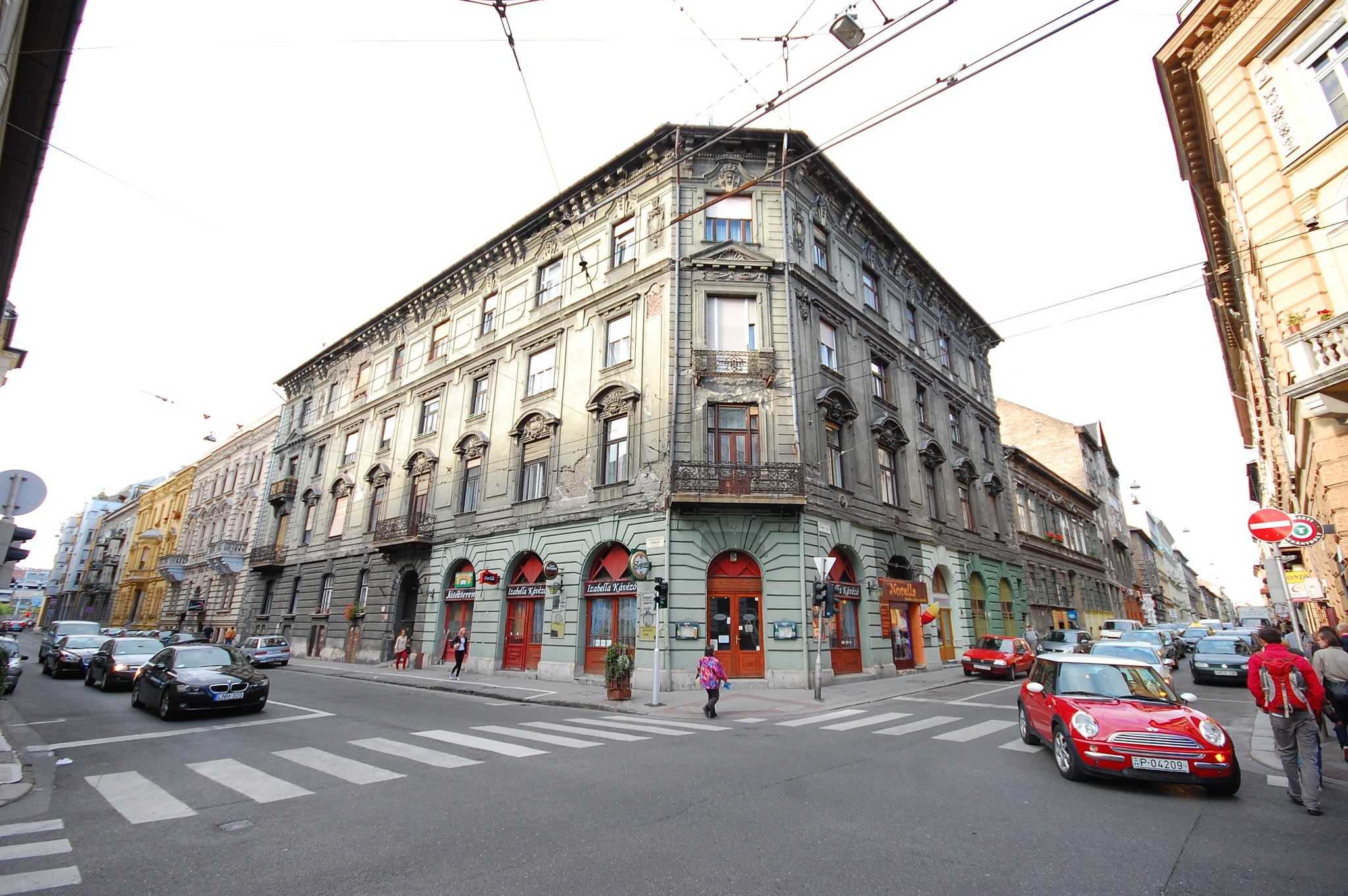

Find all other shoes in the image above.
[1287,790,1304,805]
[703,706,709,718]
[455,677,460,681]
[1308,808,1323,816]
[403,668,408,671]
[396,668,399,671]
[710,713,717,718]
[450,672,453,679]
[1343,746,1348,762]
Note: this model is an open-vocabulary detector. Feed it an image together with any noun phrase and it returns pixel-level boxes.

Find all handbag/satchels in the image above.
[695,665,702,689]
[1322,677,1348,707]
[450,635,460,650]
[406,643,411,654]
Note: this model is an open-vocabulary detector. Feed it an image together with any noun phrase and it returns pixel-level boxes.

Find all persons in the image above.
[449,627,468,681]
[696,646,728,718]
[203,624,214,640]
[1023,626,1040,656]
[224,627,236,645]
[394,628,408,671]
[1248,619,1348,816]
[0,623,6,637]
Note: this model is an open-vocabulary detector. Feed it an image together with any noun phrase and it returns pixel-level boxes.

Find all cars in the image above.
[1018,653,1241,796]
[1099,619,1263,673]
[1089,640,1175,691]
[961,635,1036,681]
[1186,634,1258,687]
[1036,629,1095,656]
[131,643,270,721]
[0,636,29,695]
[0,618,37,633]
[42,635,113,680]
[100,627,180,643]
[234,636,291,669]
[84,637,169,692]
[168,632,211,646]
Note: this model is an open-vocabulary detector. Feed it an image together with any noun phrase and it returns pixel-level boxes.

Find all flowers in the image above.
[343,603,365,621]
[1045,532,1063,543]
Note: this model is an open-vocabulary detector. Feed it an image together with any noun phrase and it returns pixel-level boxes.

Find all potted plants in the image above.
[600,642,635,701]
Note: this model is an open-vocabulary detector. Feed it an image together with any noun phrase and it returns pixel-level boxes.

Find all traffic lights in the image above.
[0,520,37,567]
[653,582,668,608]
[825,583,841,618]
[812,581,827,607]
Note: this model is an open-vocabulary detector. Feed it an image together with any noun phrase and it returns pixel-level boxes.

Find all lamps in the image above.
[868,581,883,597]
[548,580,563,595]
[1129,479,1140,489]
[203,433,217,442]
[421,589,434,601]
[1183,527,1189,532]
[829,13,865,50]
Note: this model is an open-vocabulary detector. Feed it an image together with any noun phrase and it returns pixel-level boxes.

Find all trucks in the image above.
[1235,606,1271,628]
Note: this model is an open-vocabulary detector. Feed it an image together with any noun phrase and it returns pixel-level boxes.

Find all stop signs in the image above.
[1247,508,1293,543]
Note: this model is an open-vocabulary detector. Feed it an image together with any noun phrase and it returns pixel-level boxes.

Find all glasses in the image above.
[1315,638,1324,642]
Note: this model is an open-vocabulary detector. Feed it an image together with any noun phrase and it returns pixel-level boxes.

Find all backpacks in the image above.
[1254,651,1315,720]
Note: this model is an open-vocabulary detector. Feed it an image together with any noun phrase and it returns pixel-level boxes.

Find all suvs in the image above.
[37,620,101,665]
